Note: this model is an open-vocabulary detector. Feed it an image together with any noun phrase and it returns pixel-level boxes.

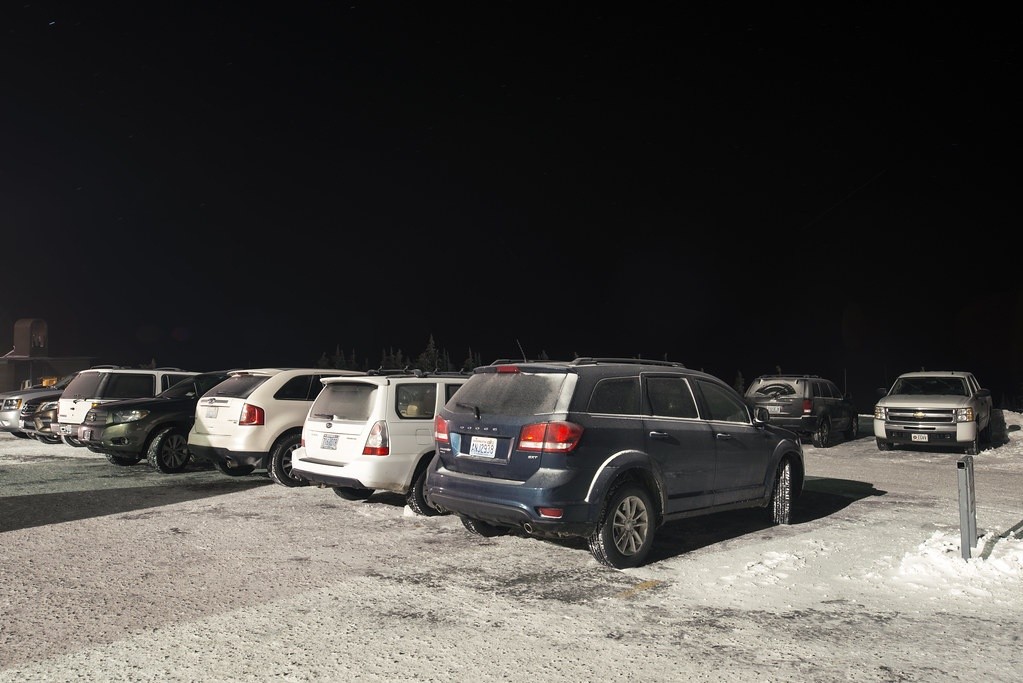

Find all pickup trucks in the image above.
[872,370,993,455]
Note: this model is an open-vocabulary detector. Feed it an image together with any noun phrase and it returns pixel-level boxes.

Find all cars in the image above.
[1,371,81,444]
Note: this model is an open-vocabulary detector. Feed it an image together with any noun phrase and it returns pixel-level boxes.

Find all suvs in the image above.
[424,352,805,568]
[50,364,205,454]
[76,367,246,474]
[188,367,431,488]
[291,370,473,517]
[744,372,859,449]
